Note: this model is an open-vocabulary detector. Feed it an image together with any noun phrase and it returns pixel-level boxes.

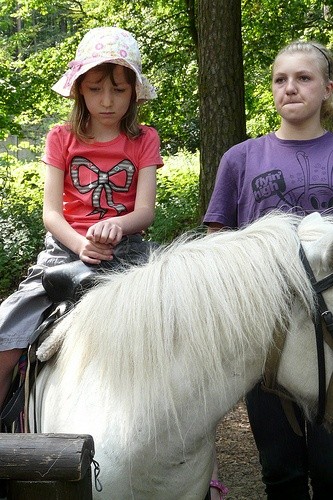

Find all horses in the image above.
[9,206,333,500]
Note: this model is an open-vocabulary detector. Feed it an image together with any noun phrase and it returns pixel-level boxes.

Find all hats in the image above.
[51,25,158,103]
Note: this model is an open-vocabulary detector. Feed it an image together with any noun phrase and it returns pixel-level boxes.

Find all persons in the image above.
[203,40,332,500]
[1,27,164,410]
